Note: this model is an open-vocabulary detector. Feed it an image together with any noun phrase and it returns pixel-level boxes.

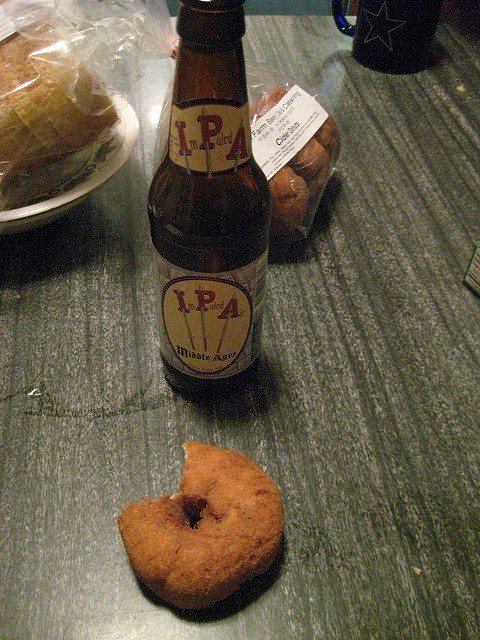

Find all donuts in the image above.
[116,441,285,610]
[251,85,341,238]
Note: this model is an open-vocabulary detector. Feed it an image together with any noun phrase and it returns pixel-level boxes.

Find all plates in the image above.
[0,90,139,234]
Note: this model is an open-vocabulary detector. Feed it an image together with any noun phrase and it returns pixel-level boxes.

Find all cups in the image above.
[331,0,444,74]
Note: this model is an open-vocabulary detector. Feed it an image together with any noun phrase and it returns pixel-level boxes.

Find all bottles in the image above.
[147,1,270,398]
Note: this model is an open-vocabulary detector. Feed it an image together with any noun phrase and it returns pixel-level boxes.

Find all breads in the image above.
[1,28,119,208]
